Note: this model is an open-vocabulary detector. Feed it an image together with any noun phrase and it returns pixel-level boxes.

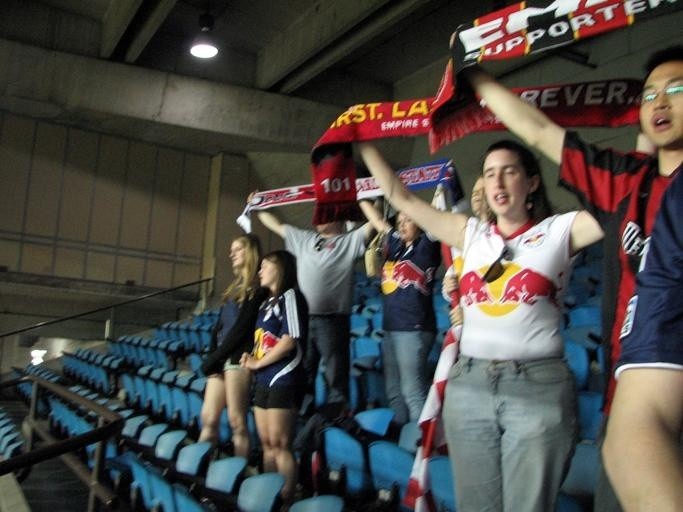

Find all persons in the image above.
[198,140,605,511]
[449,25,682,511]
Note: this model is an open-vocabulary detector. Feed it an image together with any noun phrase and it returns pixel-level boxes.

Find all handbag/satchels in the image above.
[363,246,384,279]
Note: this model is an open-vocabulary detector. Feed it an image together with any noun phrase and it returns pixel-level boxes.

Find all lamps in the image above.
[189,22,220,61]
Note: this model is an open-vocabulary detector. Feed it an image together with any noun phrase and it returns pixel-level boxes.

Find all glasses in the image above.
[481,247,514,284]
[641,81,683,103]
